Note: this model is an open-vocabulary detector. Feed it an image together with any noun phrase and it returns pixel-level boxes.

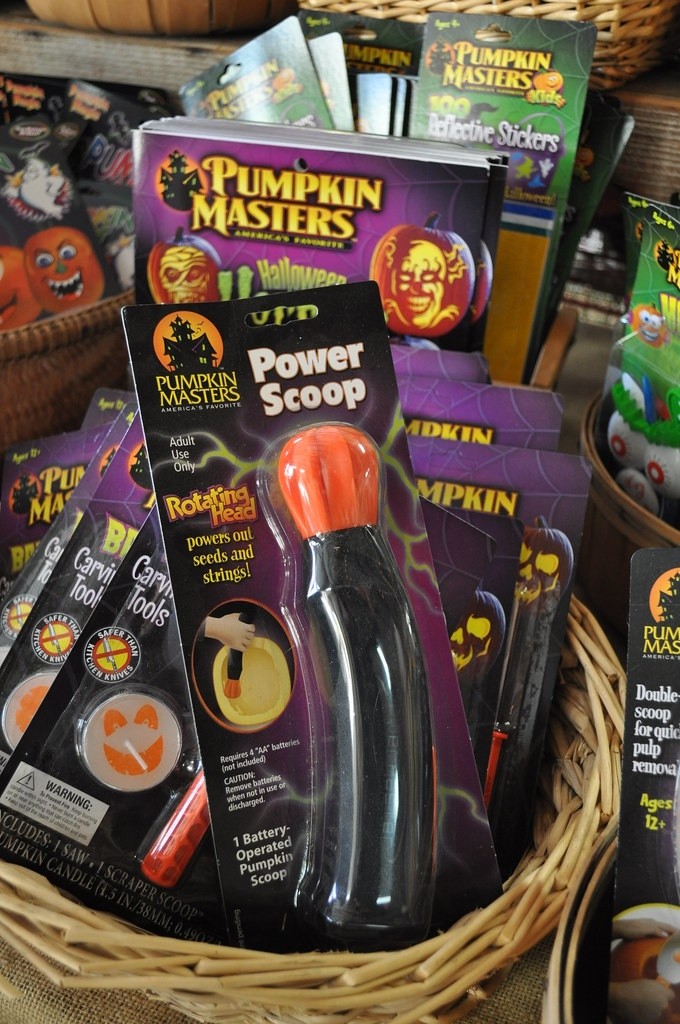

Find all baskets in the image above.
[542,813,621,1024]
[578,385,680,549]
[0,287,137,455]
[299,0,680,92]
[0,598,629,1024]
